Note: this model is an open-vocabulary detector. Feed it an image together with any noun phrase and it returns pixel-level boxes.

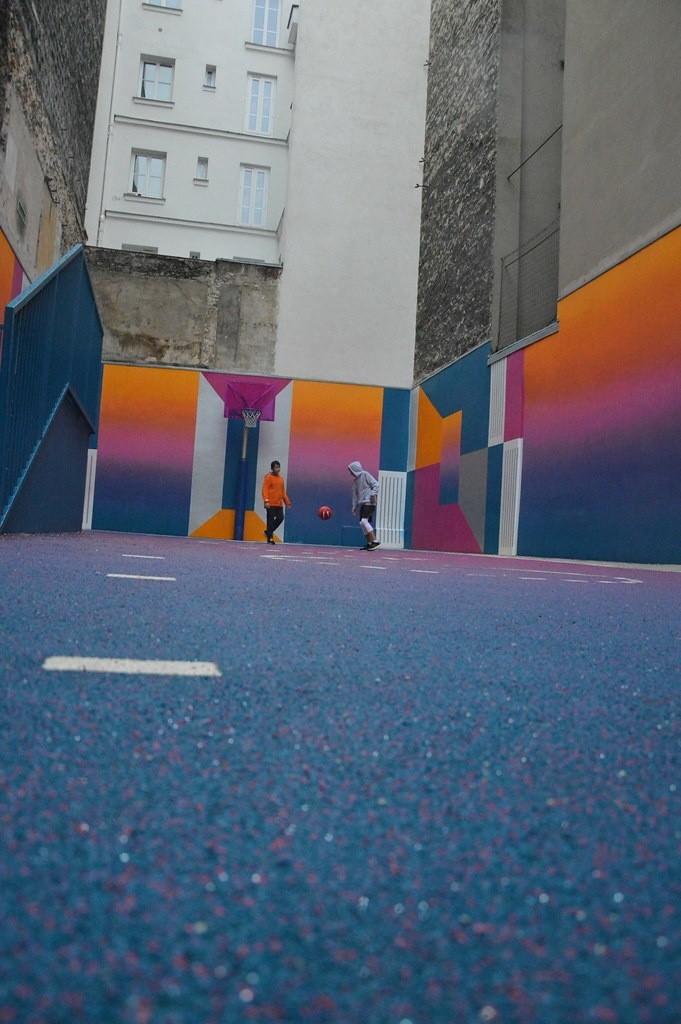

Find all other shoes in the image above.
[262,530,267,537]
[266,538,274,545]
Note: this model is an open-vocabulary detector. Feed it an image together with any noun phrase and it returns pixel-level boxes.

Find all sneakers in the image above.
[359,542,382,551]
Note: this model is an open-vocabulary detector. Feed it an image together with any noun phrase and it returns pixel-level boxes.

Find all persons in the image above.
[262,461,291,545]
[347,461,383,551]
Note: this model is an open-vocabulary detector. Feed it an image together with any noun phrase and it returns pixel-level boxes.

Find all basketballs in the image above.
[318,506,331,520]
